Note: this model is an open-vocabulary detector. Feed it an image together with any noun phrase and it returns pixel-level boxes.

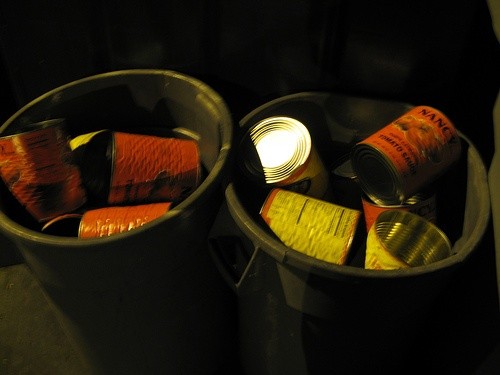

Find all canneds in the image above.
[0,103,462,269]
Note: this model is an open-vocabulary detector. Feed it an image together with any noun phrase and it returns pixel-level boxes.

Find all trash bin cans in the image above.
[224,91,491,375]
[0,70,237,375]
